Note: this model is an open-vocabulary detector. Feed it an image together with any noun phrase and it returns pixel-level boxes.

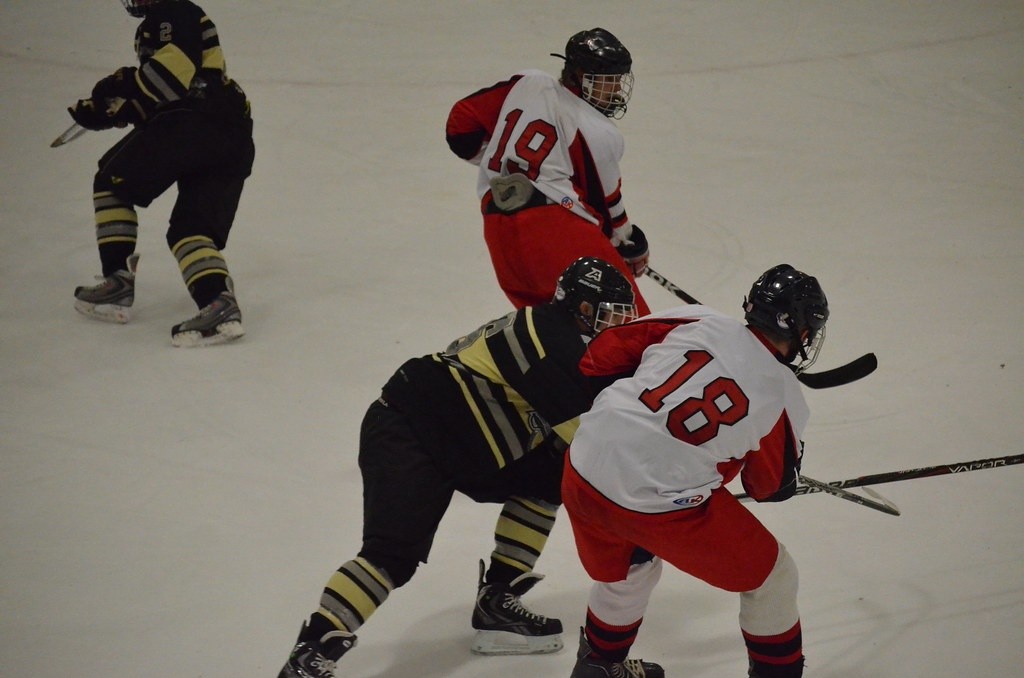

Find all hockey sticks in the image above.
[798,475,902,516]
[643,266,877,389]
[732,452,1024,500]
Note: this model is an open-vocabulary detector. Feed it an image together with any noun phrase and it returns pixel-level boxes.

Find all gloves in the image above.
[66,66,137,132]
[614,222,650,277]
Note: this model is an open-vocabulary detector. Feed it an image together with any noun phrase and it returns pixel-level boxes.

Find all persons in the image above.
[560,264,828,678]
[446,28,651,319]
[69,0,259,348]
[273,258,643,678]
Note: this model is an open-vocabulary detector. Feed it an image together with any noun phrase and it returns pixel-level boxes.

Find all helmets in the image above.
[561,28,635,116]
[552,257,640,339]
[740,263,830,375]
[123,0,162,18]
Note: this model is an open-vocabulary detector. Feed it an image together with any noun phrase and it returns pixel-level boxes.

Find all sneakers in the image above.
[274,619,360,678]
[471,559,564,656]
[570,627,663,677]
[73,253,140,325]
[171,290,244,348]
